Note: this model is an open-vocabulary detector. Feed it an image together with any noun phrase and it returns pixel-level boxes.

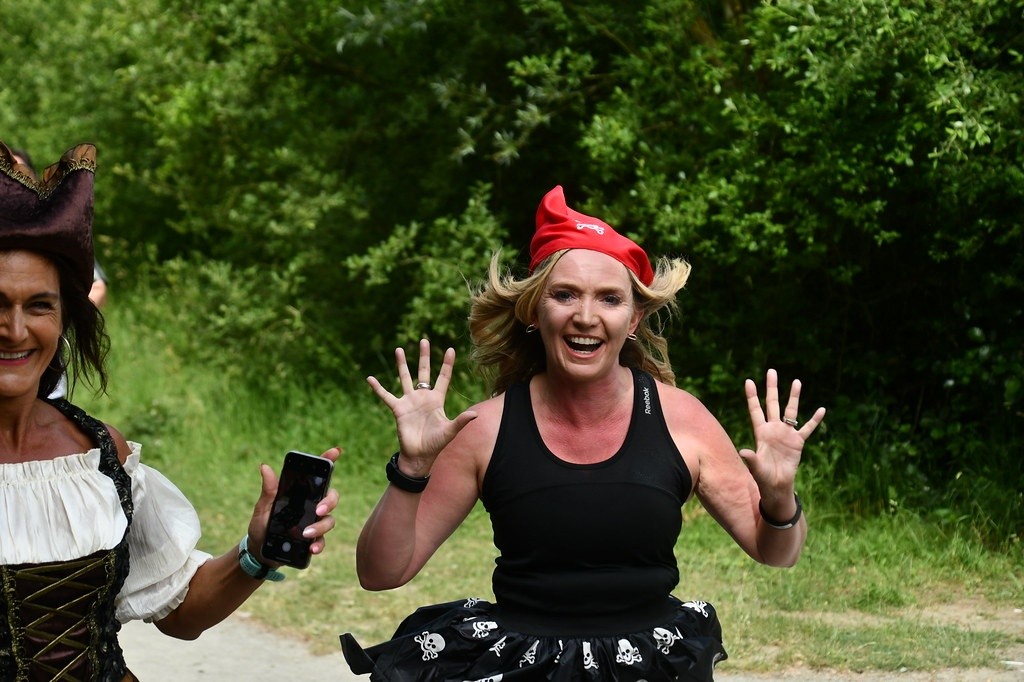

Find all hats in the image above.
[530,183,654,290]
[0,141,97,296]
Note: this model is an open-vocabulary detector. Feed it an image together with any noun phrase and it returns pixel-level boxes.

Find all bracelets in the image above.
[759,491,802,529]
[386,451,431,494]
[239,534,285,583]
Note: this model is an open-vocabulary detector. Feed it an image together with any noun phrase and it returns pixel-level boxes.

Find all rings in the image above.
[417,383,431,389]
[783,416,798,426]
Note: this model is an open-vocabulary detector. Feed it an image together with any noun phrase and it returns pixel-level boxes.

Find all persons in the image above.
[10,148,109,399]
[0,136,338,682]
[339,186,826,682]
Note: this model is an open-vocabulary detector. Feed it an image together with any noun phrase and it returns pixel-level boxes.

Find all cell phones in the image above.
[260,450,334,569]
